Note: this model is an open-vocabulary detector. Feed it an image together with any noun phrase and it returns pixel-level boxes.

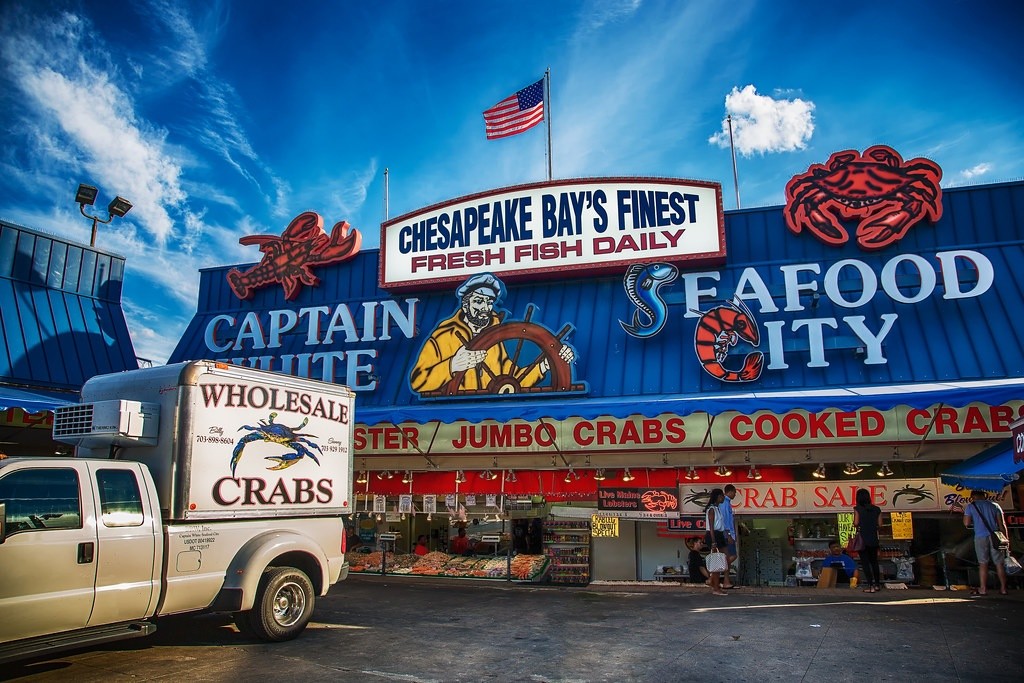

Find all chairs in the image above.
[37,469,74,514]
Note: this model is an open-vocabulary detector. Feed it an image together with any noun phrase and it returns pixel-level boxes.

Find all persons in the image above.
[345,525,363,553]
[414,536,428,556]
[450,527,472,557]
[853,488,882,593]
[964,490,1011,595]
[822,541,856,583]
[705,484,740,596]
[687,537,709,584]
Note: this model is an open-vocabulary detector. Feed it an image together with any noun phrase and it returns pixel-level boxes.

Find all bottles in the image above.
[542,520,592,585]
[881,547,901,558]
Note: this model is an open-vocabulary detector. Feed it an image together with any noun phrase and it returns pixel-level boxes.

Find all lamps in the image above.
[455,470,466,483]
[593,468,605,480]
[495,514,500,522]
[377,470,393,480]
[622,468,635,481]
[348,514,354,520]
[812,463,826,478]
[564,469,580,483]
[876,462,894,476]
[685,466,700,480]
[427,513,431,520]
[844,462,863,475]
[479,470,497,480]
[402,513,406,520]
[483,513,489,522]
[377,514,381,520]
[505,470,518,482]
[356,471,367,484]
[747,464,762,480]
[714,466,732,477]
[74,182,133,247]
[401,470,413,483]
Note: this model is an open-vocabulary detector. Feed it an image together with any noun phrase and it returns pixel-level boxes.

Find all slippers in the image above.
[969,589,988,597]
[722,584,740,590]
[996,590,1007,595]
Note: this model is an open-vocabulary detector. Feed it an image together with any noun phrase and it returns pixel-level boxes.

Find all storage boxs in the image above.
[739,518,789,588]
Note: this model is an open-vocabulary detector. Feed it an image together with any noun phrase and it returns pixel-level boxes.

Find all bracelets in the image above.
[727,532,731,536]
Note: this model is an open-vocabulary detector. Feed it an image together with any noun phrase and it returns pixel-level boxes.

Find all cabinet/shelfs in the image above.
[543,528,590,587]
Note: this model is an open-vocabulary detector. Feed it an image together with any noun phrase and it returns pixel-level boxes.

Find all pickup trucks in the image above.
[0,359,357,664]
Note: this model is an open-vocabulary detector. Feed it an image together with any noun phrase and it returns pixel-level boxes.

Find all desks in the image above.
[653,570,737,586]
[790,556,915,588]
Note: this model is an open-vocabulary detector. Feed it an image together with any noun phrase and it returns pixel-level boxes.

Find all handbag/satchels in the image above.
[706,547,728,572]
[1003,549,1022,575]
[847,527,862,552]
[990,530,1009,551]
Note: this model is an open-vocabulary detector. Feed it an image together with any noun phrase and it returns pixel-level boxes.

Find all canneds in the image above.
[550,534,589,555]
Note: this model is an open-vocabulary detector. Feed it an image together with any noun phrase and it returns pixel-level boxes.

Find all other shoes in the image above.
[713,590,728,596]
[705,580,721,589]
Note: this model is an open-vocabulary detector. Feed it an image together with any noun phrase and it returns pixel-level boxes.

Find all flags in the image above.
[483,78,544,139]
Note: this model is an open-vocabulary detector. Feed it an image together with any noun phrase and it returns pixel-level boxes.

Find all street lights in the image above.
[75,183,133,247]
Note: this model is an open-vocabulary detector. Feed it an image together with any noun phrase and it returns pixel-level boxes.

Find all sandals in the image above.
[864,585,880,593]
